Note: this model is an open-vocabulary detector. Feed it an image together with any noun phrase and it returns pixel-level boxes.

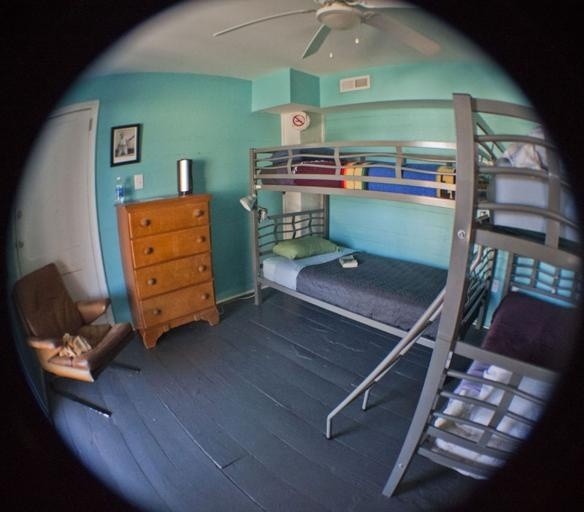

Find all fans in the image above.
[210,0,442,59]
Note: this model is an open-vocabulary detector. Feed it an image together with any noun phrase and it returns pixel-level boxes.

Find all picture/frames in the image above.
[111,123,141,167]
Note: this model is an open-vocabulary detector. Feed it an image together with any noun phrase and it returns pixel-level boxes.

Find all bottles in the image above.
[115,176,125,204]
[178,158,193,193]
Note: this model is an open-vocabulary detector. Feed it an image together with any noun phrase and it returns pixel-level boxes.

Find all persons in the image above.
[117,132,129,156]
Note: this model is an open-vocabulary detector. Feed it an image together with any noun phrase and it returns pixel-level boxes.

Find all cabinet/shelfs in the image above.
[114,193,220,349]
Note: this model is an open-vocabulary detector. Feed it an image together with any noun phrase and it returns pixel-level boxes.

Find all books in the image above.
[339,255,359,268]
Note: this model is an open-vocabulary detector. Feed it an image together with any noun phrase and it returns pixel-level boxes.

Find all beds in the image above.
[326,93,581,498]
[247,142,496,376]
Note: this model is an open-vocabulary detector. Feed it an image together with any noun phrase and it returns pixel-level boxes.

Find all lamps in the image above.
[240,193,269,225]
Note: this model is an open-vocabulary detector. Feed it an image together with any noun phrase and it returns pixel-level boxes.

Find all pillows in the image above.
[501,129,566,174]
[479,291,578,369]
[267,149,344,165]
[272,234,340,258]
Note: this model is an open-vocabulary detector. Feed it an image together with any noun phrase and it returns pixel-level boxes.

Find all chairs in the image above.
[12,262,139,420]
